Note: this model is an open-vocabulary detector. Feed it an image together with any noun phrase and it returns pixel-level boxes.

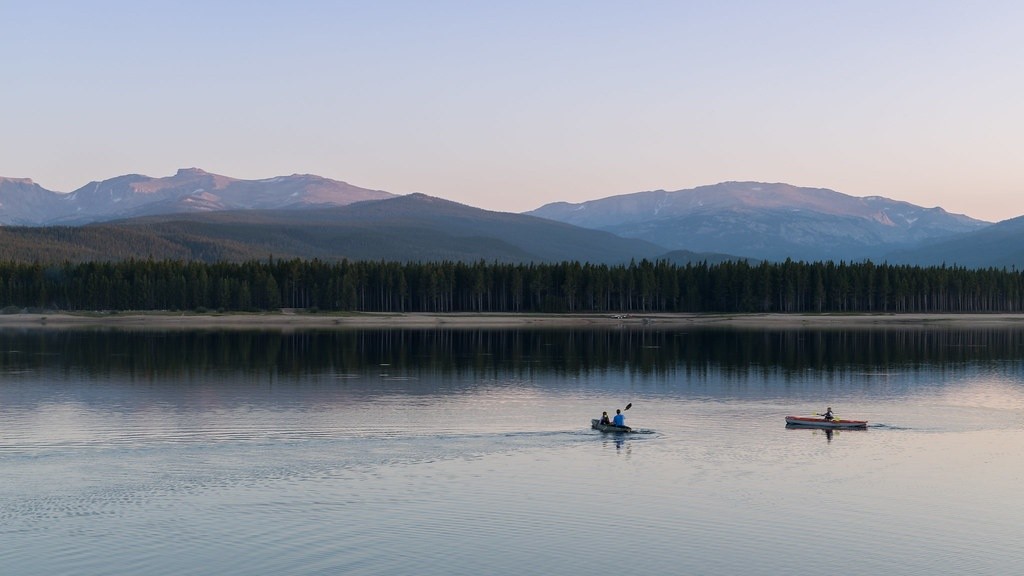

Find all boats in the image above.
[784,424,867,433]
[785,415,867,429]
[591,417,632,434]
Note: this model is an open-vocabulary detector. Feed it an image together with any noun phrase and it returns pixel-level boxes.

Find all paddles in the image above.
[602,402,633,431]
[812,411,840,422]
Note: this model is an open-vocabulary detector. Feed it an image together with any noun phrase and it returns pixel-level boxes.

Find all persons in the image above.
[599,411,610,425]
[610,409,626,427]
[821,406,835,421]
